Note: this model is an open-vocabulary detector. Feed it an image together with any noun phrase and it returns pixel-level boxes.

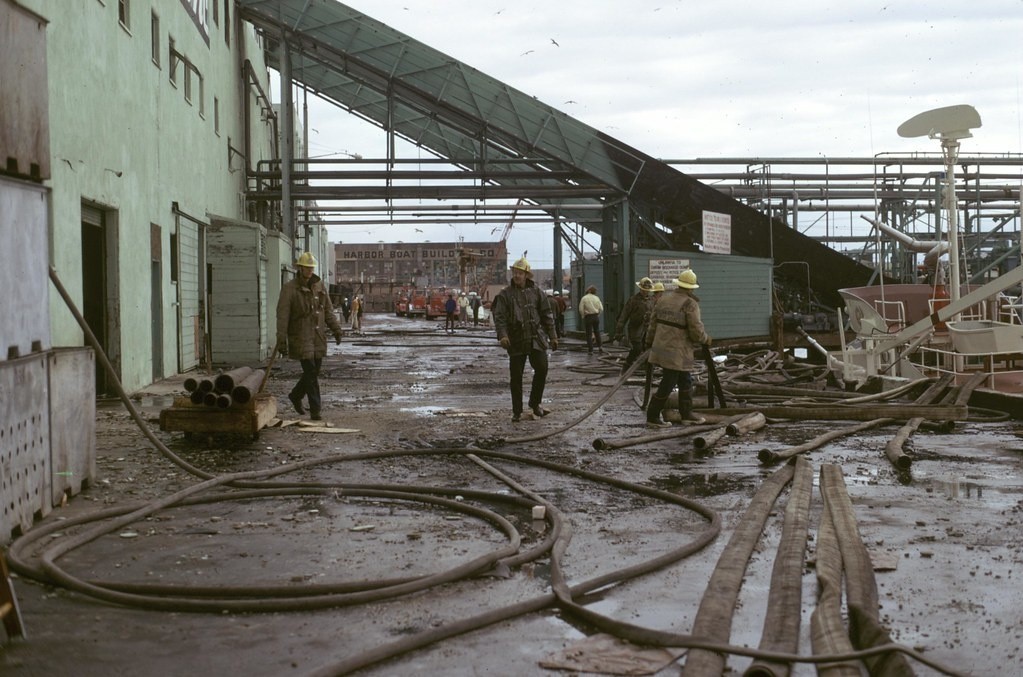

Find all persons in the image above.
[646,267,710,427]
[351,295,363,330]
[579,285,604,355]
[444,294,456,333]
[614,276,658,370]
[457,291,469,326]
[342,298,350,324]
[469,294,482,326]
[490,257,558,421]
[276,252,343,421]
[652,283,665,302]
[546,294,560,337]
[553,290,566,337]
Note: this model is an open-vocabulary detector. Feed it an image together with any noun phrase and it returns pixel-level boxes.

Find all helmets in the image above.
[652,282,666,293]
[510,260,533,278]
[636,277,652,291]
[297,252,316,267]
[672,269,700,289]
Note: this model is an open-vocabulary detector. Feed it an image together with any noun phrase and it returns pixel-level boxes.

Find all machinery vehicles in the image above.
[458,198,523,299]
[393,284,461,320]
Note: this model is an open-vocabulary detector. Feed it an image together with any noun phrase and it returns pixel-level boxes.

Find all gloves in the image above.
[501,337,511,349]
[550,339,558,351]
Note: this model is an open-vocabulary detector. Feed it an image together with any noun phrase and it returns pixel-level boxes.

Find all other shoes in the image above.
[512,414,520,421]
[310,411,322,420]
[620,364,630,377]
[528,401,543,417]
[560,332,565,337]
[598,346,603,354]
[588,352,594,355]
[289,393,306,414]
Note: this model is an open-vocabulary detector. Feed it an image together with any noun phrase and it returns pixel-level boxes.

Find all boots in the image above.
[678,389,706,426]
[646,394,671,427]
